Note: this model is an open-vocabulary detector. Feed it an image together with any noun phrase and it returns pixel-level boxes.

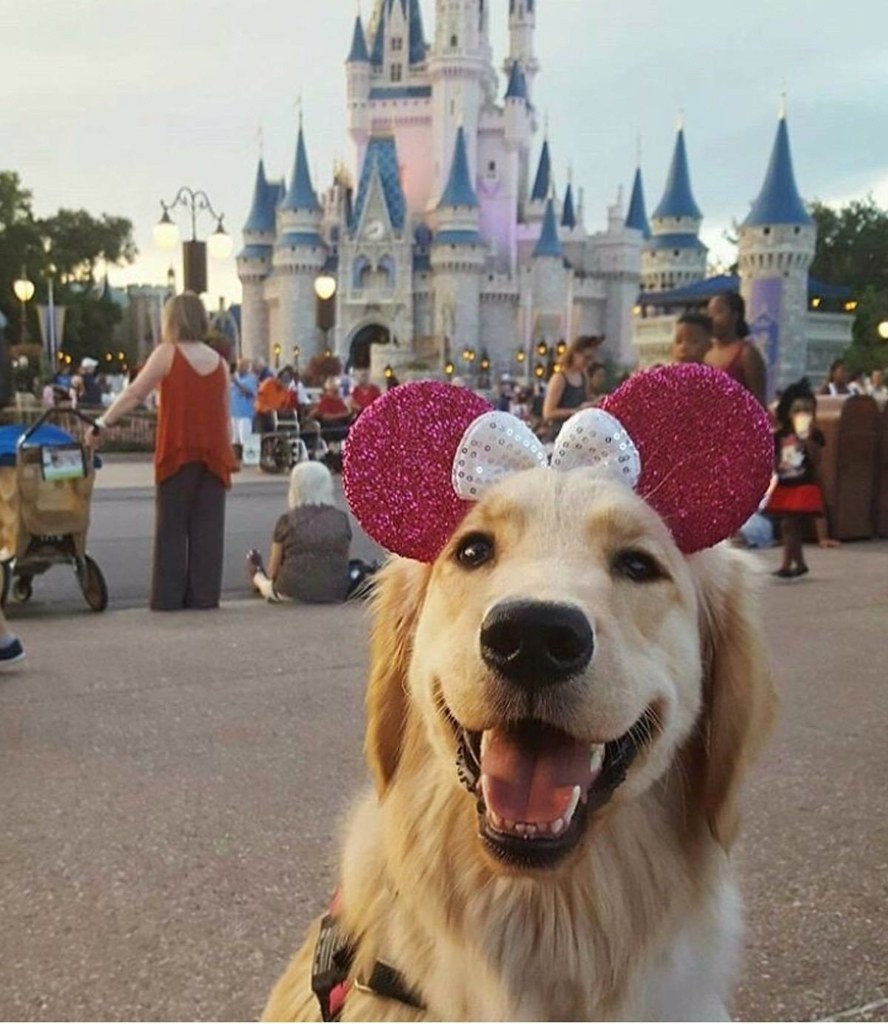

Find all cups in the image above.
[794,411,811,439]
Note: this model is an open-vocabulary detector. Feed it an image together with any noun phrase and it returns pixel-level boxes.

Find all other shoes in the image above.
[246,548,266,592]
[792,565,808,576]
[0,636,26,664]
[771,569,792,578]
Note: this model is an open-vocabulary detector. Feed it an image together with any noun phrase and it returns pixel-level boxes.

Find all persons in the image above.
[706,289,767,410]
[0,608,26,663]
[755,359,888,576]
[12,356,160,414]
[674,312,715,369]
[230,357,382,470]
[85,292,231,609]
[244,460,352,604]
[497,334,606,443]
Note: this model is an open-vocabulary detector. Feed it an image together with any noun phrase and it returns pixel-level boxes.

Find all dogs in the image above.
[258,463,773,1023]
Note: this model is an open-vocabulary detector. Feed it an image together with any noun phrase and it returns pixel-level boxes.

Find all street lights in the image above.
[11,262,36,345]
[306,266,338,355]
[40,260,60,375]
[153,185,235,298]
[272,342,283,370]
[292,345,300,383]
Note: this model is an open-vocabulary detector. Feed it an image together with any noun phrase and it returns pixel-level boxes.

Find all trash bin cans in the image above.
[767,389,888,542]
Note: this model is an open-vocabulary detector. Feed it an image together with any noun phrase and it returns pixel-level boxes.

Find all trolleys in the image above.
[0,403,109,613]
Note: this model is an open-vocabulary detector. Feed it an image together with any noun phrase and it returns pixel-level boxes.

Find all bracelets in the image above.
[93,415,112,428]
